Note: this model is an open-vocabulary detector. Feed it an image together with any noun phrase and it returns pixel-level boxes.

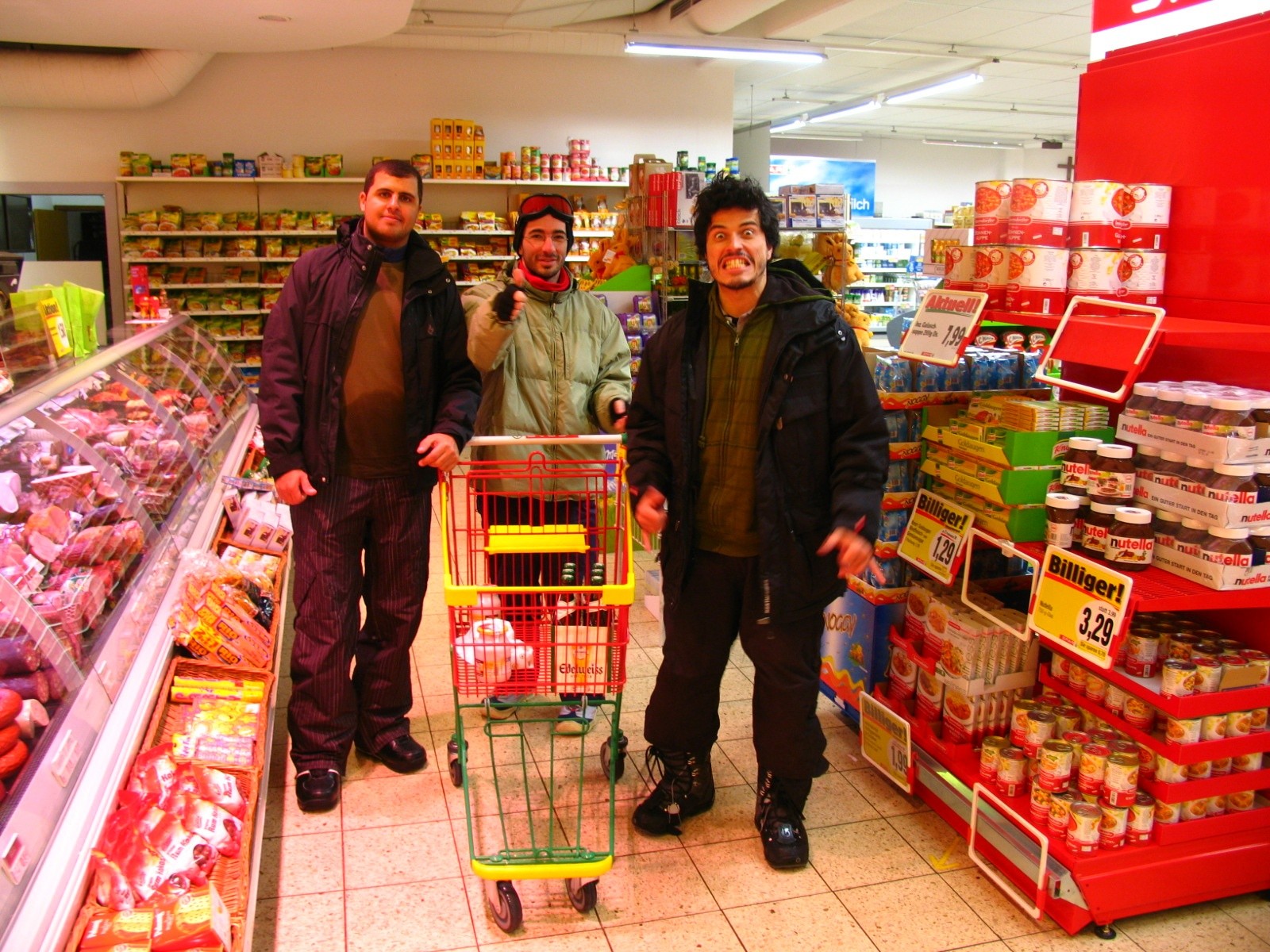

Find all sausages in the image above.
[0,505,146,807]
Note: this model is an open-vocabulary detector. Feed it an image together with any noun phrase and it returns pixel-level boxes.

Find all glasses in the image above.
[522,231,570,246]
[517,192,575,224]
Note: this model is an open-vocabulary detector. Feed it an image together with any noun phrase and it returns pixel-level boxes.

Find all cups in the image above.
[0,481,19,523]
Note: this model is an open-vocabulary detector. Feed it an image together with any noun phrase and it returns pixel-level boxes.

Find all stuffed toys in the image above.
[811,233,872,349]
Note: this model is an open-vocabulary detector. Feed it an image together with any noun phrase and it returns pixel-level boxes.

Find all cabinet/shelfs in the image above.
[115,176,1270,952]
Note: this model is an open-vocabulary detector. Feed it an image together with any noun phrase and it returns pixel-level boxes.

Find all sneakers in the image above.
[632,742,716,835]
[295,766,339,810]
[755,769,809,869]
[379,735,427,773]
[480,686,536,721]
[556,701,596,734]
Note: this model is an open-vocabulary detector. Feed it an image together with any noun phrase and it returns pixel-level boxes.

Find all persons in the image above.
[462,191,634,733]
[255,159,484,812]
[625,176,889,869]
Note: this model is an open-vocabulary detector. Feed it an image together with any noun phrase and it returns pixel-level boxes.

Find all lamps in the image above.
[625,0,827,66]
[769,67,984,134]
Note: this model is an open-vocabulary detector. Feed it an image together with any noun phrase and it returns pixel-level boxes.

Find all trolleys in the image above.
[435,430,644,935]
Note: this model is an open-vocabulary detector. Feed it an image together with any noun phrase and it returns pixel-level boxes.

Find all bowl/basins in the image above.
[31,471,94,510]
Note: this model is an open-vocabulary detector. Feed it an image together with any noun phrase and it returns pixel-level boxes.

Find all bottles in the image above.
[953,207,974,229]
[583,562,611,692]
[551,562,581,692]
[475,623,512,683]
[469,584,501,628]
[931,239,959,263]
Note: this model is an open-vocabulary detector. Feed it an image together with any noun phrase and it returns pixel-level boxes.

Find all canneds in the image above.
[214,152,235,177]
[471,584,511,682]
[500,138,630,183]
[1043,382,1270,571]
[674,150,739,184]
[978,609,1270,854]
